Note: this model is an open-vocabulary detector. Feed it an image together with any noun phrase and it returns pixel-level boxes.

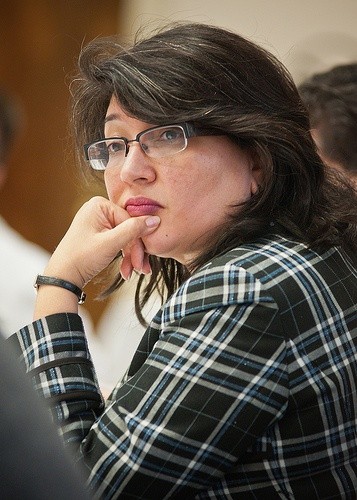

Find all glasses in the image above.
[83,120,227,172]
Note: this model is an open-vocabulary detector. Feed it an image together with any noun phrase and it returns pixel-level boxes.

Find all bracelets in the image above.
[33,275,87,305]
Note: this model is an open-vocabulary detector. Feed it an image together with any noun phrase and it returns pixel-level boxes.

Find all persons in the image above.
[291,61,356,183]
[7,16,356,500]
[0,101,95,333]
